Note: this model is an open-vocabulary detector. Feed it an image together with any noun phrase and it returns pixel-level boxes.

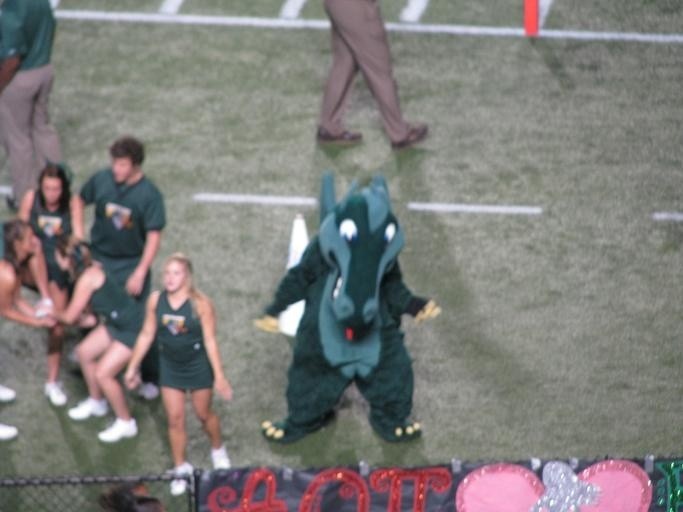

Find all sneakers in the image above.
[391,122,427,147]
[67,395,110,420]
[134,383,161,401]
[97,416,141,443]
[0,384,19,442]
[42,381,69,407]
[316,124,362,142]
[209,443,233,472]
[169,462,194,496]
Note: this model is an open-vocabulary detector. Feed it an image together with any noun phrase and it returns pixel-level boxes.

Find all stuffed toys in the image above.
[250,156,445,443]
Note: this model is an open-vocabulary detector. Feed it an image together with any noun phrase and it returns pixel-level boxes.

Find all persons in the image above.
[17,163,93,409]
[314,0,428,150]
[65,133,168,403]
[32,230,144,445]
[123,248,236,498]
[0,218,58,446]
[0,0,72,212]
[96,478,167,512]
